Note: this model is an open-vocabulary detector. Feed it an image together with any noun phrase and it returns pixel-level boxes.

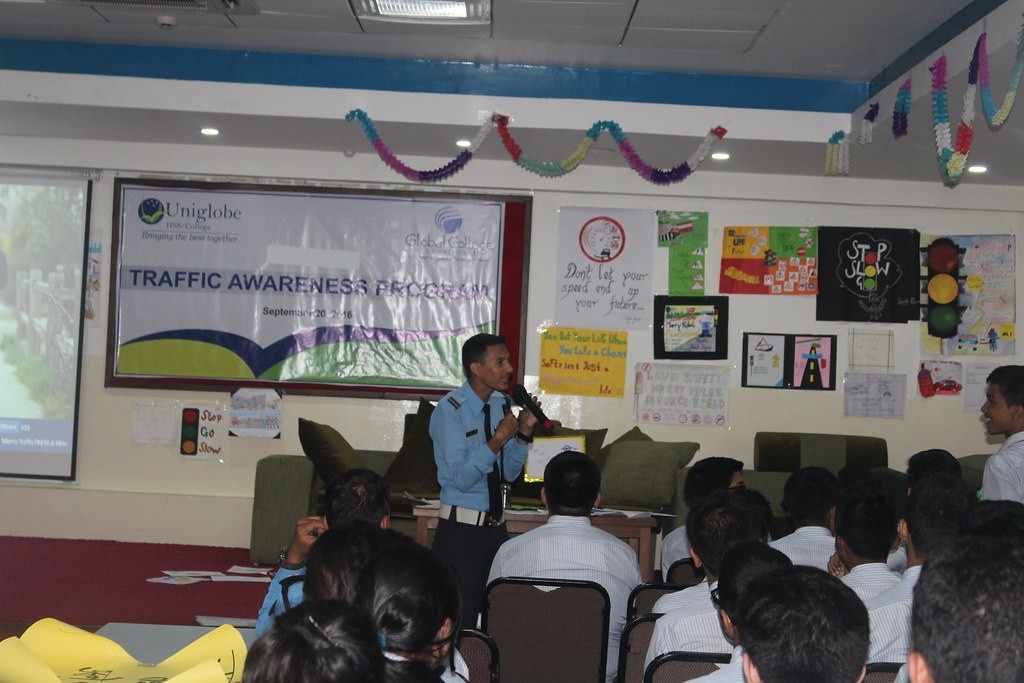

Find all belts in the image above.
[438,503,505,526]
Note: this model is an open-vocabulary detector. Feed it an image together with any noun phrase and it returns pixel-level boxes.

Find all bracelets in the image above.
[278,544,306,569]
[516,431,533,443]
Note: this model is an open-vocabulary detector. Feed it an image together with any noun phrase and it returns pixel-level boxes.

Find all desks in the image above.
[96,622,256,664]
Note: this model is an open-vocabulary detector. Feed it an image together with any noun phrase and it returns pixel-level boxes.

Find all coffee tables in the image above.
[412,508,658,583]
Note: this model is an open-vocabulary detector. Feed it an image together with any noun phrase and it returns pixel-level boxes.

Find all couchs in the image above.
[662,432,907,543]
[956,453,993,490]
[250,449,663,584]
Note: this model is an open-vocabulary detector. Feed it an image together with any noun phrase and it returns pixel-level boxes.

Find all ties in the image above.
[482,403,503,523]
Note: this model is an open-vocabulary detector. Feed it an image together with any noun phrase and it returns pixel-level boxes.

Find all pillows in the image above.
[598,441,700,509]
[299,417,368,483]
[383,396,441,494]
[511,422,608,500]
[596,426,653,472]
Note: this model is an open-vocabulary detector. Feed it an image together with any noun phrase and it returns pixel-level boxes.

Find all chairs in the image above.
[481,576,611,683]
[643,651,731,683]
[617,613,666,683]
[626,583,698,623]
[457,626,501,683]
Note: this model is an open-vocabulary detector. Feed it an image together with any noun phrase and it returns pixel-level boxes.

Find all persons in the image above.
[429,334,542,629]
[864,489,1024,683]
[980,365,1024,507]
[838,463,910,533]
[475,451,644,683]
[651,497,760,613]
[827,497,902,602]
[766,466,837,576]
[242,520,470,683]
[254,467,391,636]
[660,456,772,584]
[644,540,870,683]
[906,449,961,501]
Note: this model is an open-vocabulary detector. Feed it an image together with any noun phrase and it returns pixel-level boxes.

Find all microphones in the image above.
[511,384,554,432]
